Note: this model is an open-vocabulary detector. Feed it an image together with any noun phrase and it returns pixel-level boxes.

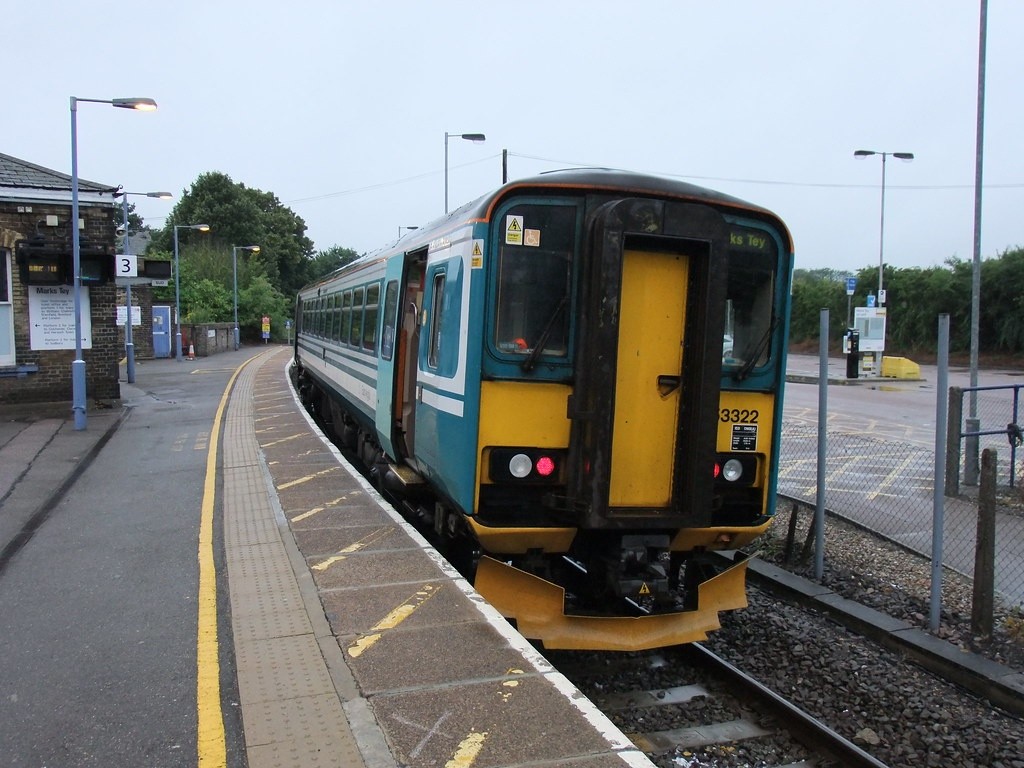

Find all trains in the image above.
[290,163,796,652]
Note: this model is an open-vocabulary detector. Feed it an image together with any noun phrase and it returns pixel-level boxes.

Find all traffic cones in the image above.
[186,339,198,360]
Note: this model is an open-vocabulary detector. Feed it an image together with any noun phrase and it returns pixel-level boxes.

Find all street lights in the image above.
[122,190,175,383]
[853,149,915,377]
[233,246,262,351]
[443,130,486,216]
[398,226,418,238]
[68,94,160,432]
[173,223,210,361]
